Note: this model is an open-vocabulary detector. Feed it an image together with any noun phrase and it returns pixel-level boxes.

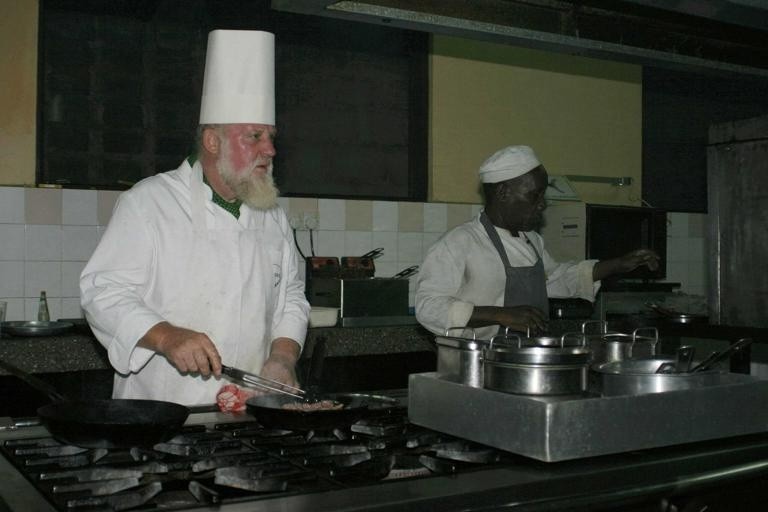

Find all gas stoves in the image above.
[0,406,499,511]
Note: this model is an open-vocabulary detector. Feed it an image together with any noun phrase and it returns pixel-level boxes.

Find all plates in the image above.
[0,320,76,335]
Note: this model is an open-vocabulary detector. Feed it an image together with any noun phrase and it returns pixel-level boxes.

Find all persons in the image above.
[415,142,661,341]
[75,123,311,410]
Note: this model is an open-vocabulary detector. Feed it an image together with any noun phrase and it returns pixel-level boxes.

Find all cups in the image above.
[0,301,8,322]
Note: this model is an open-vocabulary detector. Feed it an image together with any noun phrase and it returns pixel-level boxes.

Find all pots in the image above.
[234,385,400,429]
[1,359,191,450]
[432,307,726,400]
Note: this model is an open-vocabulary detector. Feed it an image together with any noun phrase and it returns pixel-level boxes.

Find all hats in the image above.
[479,143,543,186]
[199,28,279,126]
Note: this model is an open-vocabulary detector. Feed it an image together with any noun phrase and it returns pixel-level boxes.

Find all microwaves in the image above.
[536,202,669,283]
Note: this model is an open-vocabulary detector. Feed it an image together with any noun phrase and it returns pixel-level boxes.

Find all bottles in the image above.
[36,290,51,320]
